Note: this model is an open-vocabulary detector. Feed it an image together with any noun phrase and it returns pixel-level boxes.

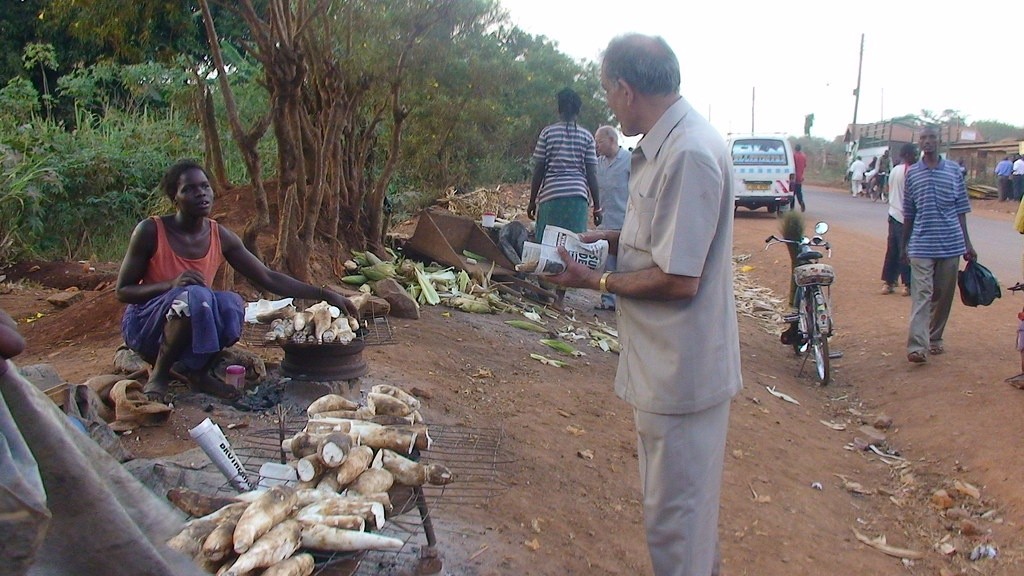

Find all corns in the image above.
[341,249,393,294]
[450,296,495,313]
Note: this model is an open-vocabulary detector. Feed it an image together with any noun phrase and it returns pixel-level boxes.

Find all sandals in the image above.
[594,303,614,311]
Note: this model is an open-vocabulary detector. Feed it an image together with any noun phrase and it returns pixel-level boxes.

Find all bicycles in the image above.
[1007,283,1024,375]
[764,222,844,385]
[869,171,890,203]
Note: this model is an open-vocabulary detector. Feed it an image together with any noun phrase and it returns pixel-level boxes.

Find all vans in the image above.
[728,135,798,216]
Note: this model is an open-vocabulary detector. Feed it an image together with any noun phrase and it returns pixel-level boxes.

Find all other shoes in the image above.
[144,375,168,403]
[880,285,895,294]
[908,352,927,362]
[192,374,235,397]
[902,287,911,296]
[930,347,944,355]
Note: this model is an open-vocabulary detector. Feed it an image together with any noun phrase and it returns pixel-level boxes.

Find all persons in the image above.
[527,88,602,312]
[590,126,632,310]
[115,159,357,404]
[540,33,743,576]
[959,157,967,179]
[900,126,977,362]
[790,144,806,212]
[995,154,1024,201]
[881,143,918,296]
[850,150,893,198]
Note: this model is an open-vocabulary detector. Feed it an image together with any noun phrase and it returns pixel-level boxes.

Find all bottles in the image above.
[226,365,245,391]
[481,212,496,228]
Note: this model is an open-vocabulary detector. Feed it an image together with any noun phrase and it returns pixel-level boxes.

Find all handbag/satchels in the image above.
[958,261,1001,307]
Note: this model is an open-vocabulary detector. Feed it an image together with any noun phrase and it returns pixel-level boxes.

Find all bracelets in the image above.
[320,284,324,296]
[592,208,602,213]
[600,271,612,293]
